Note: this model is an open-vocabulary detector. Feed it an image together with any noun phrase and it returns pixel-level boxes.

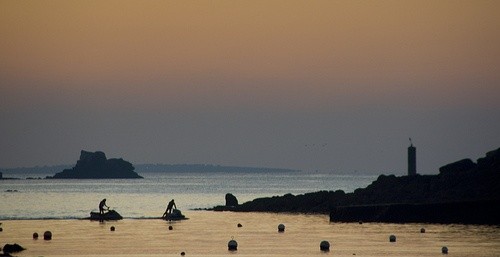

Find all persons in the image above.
[98,198,110,223]
[162,198,176,222]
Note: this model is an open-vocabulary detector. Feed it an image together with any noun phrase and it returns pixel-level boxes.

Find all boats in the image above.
[89,210,122,221]
[158,209,187,221]
